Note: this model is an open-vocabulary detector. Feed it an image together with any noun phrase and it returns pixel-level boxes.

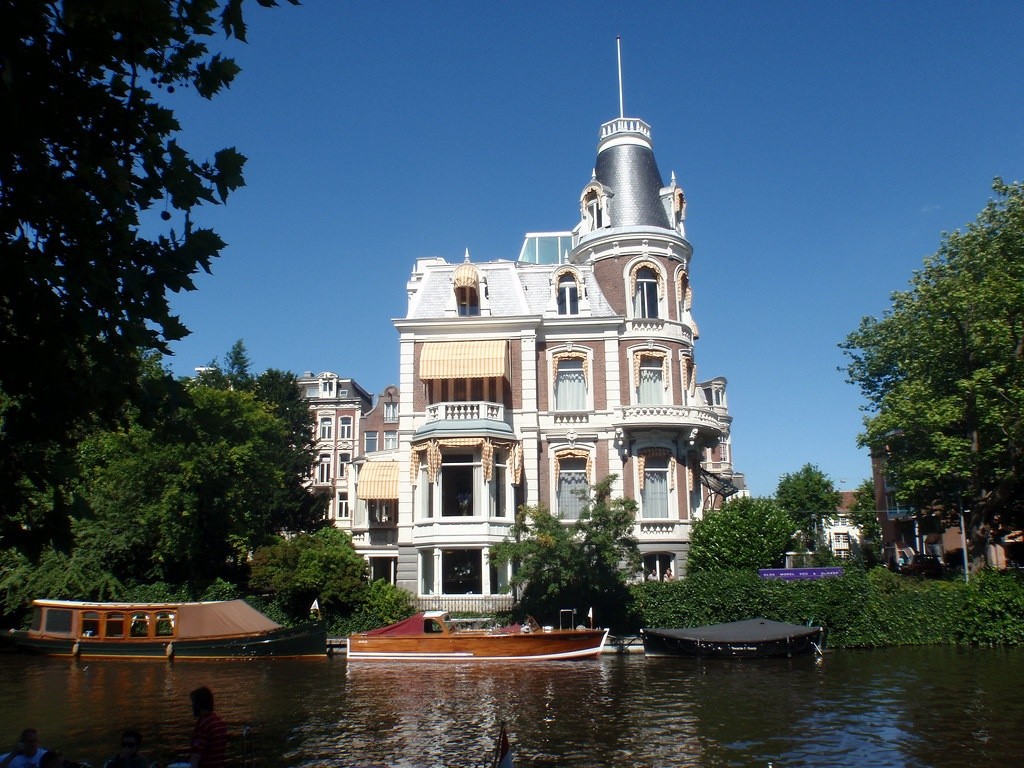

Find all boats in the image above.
[347,610,610,663]
[0,598,332,659]
[639,615,828,660]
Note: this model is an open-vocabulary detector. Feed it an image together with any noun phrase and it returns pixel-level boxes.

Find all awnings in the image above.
[701,467,739,501]
[453,266,479,297]
[357,460,399,500]
[419,340,510,383]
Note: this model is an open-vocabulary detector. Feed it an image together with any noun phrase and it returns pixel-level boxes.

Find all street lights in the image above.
[959,508,971,585]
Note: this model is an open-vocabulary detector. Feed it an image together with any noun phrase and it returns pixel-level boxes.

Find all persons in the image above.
[167,686,228,768]
[105,729,160,768]
[648,569,658,580]
[899,556,904,574]
[0,727,95,768]
[663,568,676,583]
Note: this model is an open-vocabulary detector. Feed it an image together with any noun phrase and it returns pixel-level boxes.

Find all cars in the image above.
[999,561,1024,573]
[898,554,941,575]
